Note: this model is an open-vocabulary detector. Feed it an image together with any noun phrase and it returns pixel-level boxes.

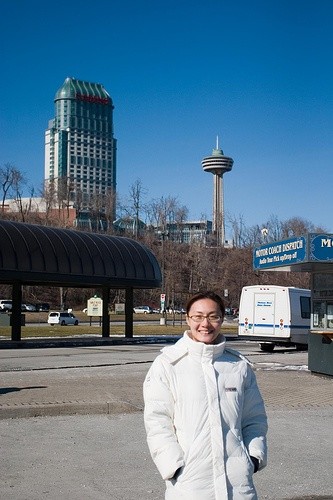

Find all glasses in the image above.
[188,315,223,322]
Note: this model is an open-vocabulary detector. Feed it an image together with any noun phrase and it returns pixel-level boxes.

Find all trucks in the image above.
[237,284,311,352]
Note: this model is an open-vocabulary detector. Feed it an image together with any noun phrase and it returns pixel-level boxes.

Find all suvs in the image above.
[0,300,12,312]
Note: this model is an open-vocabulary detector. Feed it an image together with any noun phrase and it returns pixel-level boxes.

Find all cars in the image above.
[153,308,187,314]
[225,307,239,318]
[73,208,108,231]
[83,308,88,313]
[133,305,153,314]
[21,304,37,312]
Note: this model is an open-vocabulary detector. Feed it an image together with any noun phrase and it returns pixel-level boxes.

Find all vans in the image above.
[47,312,79,326]
[35,302,50,312]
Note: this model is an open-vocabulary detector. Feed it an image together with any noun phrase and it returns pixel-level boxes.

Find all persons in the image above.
[142,291,268,500]
